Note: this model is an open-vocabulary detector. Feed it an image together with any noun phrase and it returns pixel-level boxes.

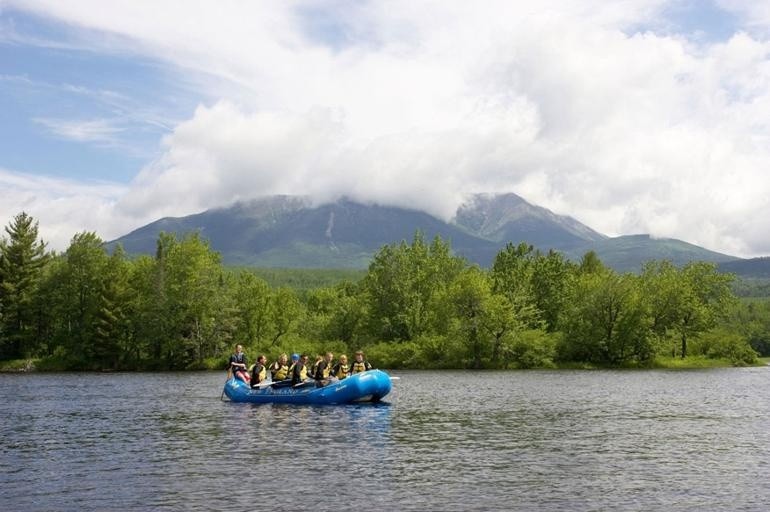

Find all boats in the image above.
[224,369,392,405]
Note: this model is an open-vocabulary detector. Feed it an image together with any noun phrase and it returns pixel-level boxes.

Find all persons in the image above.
[250,349,373,390]
[230,343,251,384]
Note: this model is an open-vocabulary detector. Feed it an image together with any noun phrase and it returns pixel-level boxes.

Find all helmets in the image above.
[292,354,299,360]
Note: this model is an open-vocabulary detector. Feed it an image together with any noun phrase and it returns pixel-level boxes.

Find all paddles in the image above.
[253,379,285,387]
[293,379,315,388]
